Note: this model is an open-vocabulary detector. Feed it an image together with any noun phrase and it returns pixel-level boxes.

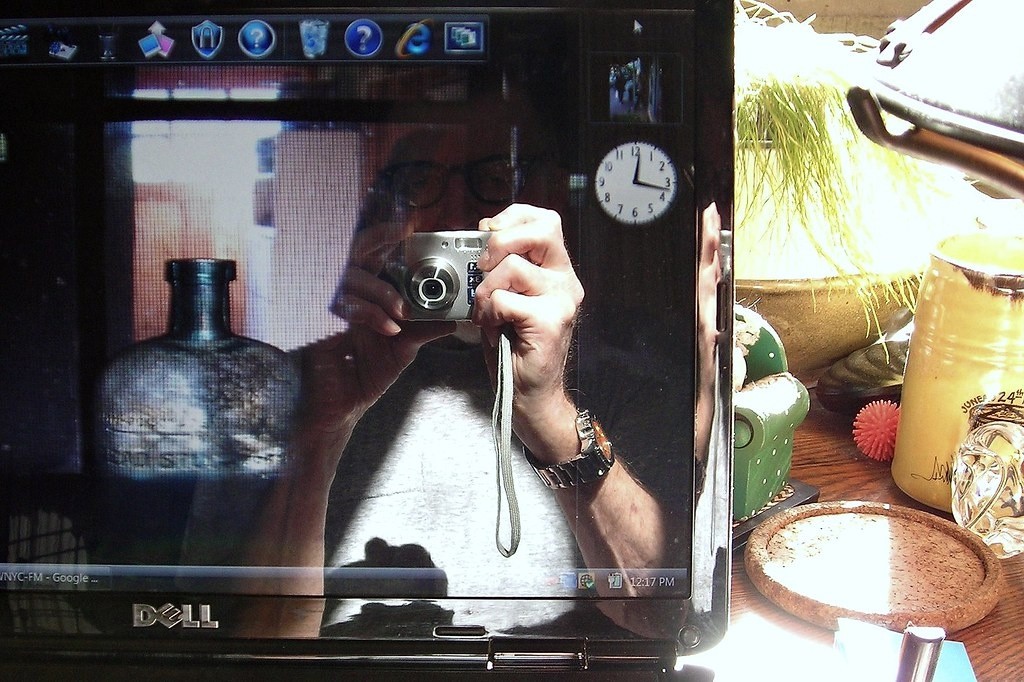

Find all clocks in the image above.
[594,140,680,227]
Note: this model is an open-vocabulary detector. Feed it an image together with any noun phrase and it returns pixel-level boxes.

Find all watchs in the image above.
[522,408,616,490]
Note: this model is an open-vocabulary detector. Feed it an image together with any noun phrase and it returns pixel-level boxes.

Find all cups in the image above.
[892,236,1023,513]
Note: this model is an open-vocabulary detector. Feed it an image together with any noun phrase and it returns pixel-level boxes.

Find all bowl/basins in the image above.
[734,267,922,371]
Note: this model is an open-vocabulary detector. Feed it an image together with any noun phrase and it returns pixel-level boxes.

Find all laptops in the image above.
[0,0,737,682]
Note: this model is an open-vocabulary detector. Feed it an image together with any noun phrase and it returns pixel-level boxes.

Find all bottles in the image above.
[89,260,302,567]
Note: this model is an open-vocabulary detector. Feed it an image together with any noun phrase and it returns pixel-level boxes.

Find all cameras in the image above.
[398,231,527,320]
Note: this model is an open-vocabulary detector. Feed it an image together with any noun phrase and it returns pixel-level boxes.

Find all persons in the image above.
[227,117,684,644]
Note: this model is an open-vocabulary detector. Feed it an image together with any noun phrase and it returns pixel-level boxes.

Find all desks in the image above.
[672,380,1024,682]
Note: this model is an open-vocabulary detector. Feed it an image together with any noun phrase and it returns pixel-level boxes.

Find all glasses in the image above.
[377,150,555,208]
